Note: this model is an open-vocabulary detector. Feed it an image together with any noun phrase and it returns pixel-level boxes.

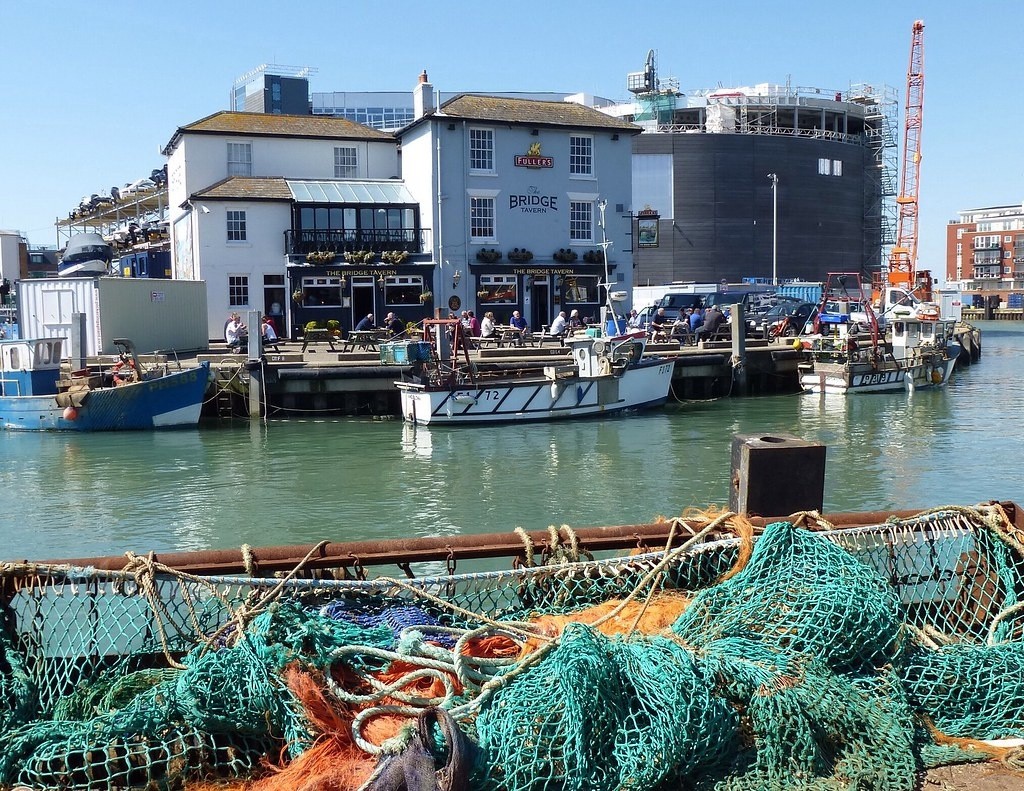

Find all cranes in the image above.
[873,17,935,304]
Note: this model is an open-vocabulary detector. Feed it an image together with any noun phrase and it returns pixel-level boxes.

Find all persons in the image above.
[423,310,481,350]
[384,311,404,337]
[355,313,379,350]
[626,305,731,347]
[550,309,586,347]
[480,310,527,348]
[224,311,279,354]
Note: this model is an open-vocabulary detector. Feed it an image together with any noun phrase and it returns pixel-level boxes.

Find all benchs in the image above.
[222,328,764,353]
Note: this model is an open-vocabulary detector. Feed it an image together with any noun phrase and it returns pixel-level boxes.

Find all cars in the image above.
[751,300,830,336]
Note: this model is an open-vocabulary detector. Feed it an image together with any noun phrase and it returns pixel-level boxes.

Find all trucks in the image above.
[851,288,925,324]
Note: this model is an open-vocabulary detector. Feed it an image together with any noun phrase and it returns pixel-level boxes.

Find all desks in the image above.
[410,330,437,336]
[342,331,380,353]
[301,329,336,353]
[664,324,695,347]
[537,323,605,348]
[476,325,527,355]
[703,319,733,341]
[240,333,267,354]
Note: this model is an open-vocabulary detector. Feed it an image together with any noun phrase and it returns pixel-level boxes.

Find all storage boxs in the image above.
[820,312,851,324]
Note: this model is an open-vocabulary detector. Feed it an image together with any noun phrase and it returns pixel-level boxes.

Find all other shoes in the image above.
[692,343,697,345]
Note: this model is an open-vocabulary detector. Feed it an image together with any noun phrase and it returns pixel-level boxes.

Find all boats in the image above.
[114,218,169,243]
[0,328,210,431]
[801,312,962,395]
[67,187,120,213]
[392,197,678,425]
[119,179,156,198]
[58,235,112,277]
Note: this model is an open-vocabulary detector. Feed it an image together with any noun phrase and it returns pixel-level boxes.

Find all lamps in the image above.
[452,269,461,289]
[340,274,347,293]
[610,134,619,142]
[377,274,385,294]
[531,129,538,136]
[447,124,456,131]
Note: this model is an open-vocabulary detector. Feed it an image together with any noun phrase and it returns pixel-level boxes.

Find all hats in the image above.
[571,309,578,317]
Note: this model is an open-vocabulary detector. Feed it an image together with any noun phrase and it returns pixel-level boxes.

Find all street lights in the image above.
[767,172,779,285]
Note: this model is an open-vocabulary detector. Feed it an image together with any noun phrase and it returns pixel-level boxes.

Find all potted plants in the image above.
[418,280,433,303]
[291,280,306,303]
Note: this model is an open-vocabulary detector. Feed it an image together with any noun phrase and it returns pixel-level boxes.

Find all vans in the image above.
[654,293,706,329]
[699,291,775,328]
